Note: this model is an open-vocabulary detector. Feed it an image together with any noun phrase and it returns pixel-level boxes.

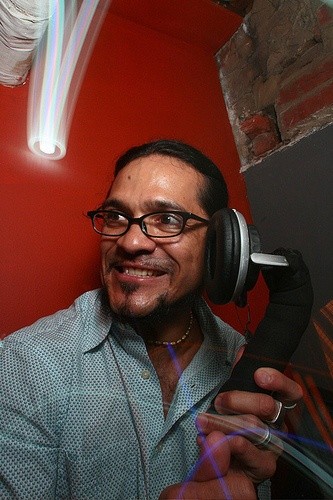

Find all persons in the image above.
[0,139,307,500]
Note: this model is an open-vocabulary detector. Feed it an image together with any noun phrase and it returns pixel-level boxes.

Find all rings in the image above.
[284,403,297,410]
[254,424,272,449]
[264,401,286,430]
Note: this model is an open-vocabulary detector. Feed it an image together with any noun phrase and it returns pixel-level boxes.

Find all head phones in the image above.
[198,209,315,439]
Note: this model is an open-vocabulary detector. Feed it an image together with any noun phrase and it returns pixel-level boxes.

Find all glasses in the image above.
[87,206,210,238]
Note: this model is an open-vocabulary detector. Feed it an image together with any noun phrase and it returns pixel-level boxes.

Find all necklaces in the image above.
[142,309,194,346]
